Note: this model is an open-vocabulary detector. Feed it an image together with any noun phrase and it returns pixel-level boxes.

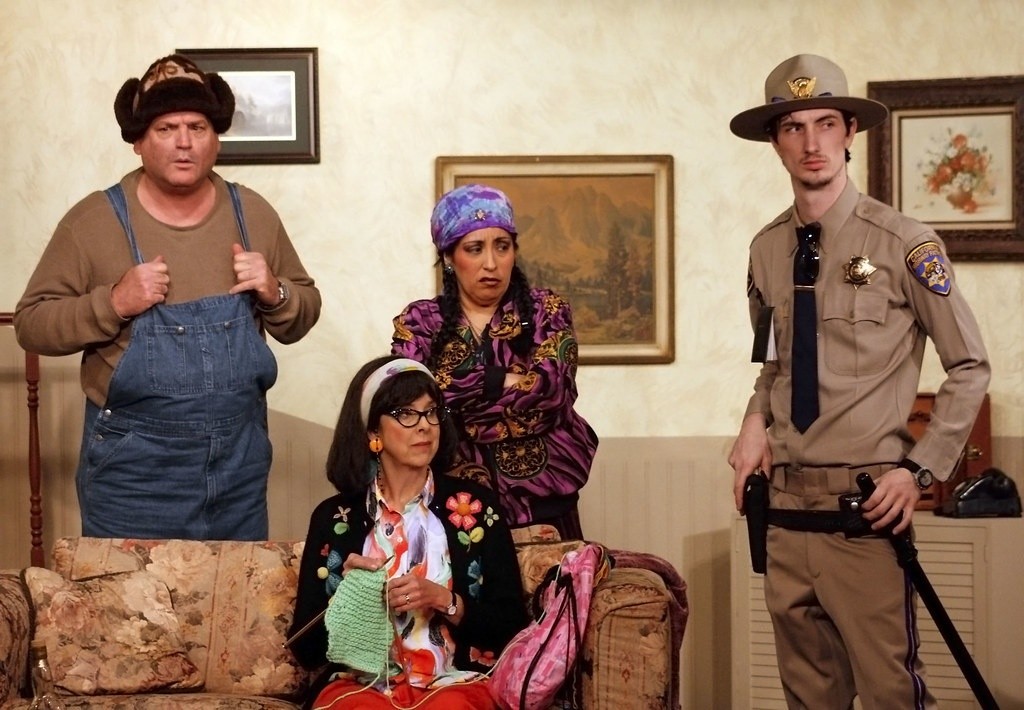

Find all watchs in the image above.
[444,591,457,616]
[274,282,285,308]
[897,458,934,490]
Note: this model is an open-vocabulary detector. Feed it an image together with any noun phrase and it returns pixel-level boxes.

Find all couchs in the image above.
[0,534,689,710]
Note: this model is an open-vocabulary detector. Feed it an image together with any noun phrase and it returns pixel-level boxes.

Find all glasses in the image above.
[384,406,451,428]
[798,224,819,280]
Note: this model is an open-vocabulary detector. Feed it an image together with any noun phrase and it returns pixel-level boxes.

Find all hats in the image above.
[729,54,889,142]
[115,55,236,143]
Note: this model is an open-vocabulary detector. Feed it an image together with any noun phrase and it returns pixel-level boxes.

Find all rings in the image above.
[405,595,409,604]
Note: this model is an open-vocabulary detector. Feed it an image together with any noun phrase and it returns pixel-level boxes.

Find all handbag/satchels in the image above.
[488,543,612,710]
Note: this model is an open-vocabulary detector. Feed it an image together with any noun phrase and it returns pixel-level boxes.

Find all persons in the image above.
[390,183,599,542]
[13,54,321,539]
[285,355,533,710]
[727,55,992,710]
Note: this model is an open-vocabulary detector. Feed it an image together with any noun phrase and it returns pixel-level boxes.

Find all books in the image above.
[751,307,778,362]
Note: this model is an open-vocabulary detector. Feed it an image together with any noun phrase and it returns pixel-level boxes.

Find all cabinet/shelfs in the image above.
[730,513,1024,710]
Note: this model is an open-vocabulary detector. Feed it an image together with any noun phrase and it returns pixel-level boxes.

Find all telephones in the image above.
[951,467,1022,517]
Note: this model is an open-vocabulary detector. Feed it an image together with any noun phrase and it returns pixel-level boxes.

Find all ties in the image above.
[791,223,821,435]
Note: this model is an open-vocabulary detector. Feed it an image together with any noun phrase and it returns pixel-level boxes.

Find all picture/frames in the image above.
[866,74,1024,263]
[175,48,321,166]
[434,156,677,365]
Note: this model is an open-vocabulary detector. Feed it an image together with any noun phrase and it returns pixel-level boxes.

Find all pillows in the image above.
[20,525,584,696]
